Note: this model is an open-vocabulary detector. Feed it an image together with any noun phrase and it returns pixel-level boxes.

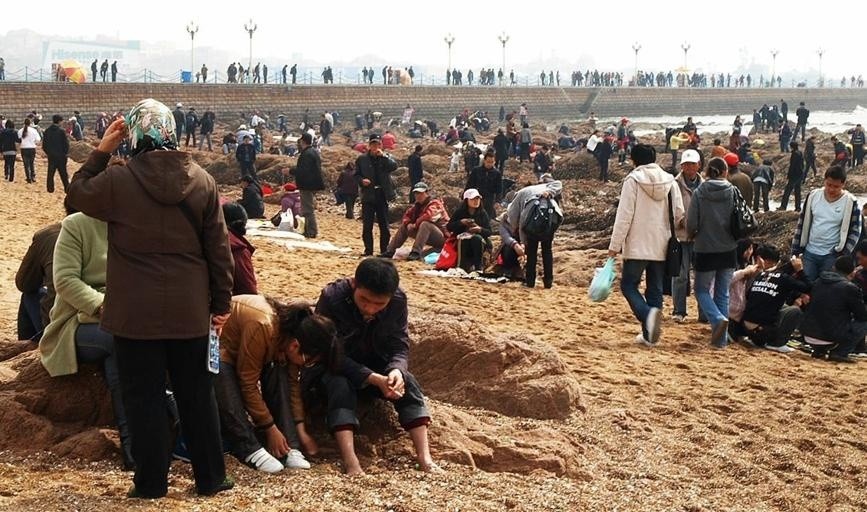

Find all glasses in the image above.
[295,338,318,368]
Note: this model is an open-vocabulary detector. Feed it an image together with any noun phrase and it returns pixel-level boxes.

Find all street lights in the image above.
[185,19,198,82]
[243,17,258,83]
[680,39,692,86]
[444,31,456,84]
[497,30,510,86]
[631,40,644,87]
[770,45,780,86]
[815,44,827,87]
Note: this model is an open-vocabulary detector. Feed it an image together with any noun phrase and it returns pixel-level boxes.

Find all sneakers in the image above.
[754,206,801,212]
[713,319,867,364]
[635,307,689,348]
[361,250,395,260]
[172,440,192,464]
[406,251,421,261]
[196,477,235,497]
[244,447,284,475]
[127,483,168,500]
[619,160,629,165]
[279,447,312,471]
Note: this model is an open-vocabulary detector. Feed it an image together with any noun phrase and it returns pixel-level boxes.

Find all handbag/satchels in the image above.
[710,265,758,321]
[335,191,345,205]
[731,201,759,239]
[520,201,553,242]
[436,240,459,271]
[664,236,684,277]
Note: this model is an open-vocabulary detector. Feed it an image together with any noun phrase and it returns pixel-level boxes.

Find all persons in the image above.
[486,99,866,363]
[0,58,5,79]
[100,59,108,82]
[213,293,339,474]
[38,210,231,468]
[111,61,117,83]
[304,257,446,476]
[91,59,97,82]
[196,61,865,89]
[64,98,235,500]
[0,99,496,363]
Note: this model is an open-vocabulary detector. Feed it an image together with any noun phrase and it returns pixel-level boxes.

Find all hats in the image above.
[301,132,313,141]
[229,111,264,137]
[176,102,183,108]
[680,149,701,166]
[369,133,383,145]
[622,119,629,123]
[724,153,740,166]
[283,183,297,191]
[413,181,430,193]
[464,188,483,200]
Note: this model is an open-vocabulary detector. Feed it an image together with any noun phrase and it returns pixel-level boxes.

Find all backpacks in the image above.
[187,114,196,128]
